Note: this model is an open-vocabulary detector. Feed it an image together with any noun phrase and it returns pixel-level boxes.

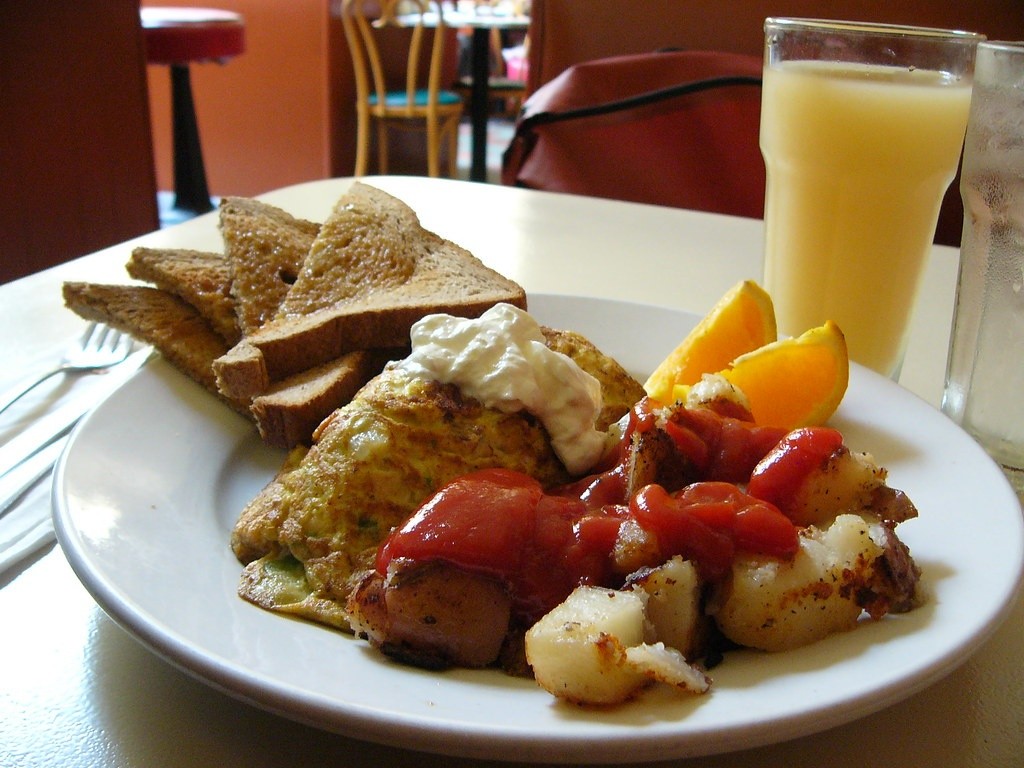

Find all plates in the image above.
[38,294,1024,759]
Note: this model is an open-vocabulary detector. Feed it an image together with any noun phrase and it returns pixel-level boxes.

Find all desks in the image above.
[134,6,250,214]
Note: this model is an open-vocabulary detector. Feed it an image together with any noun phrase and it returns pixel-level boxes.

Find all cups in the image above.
[940,40,1024,498]
[759,17,985,383]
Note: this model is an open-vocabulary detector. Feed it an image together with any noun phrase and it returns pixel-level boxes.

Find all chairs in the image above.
[335,0,473,182]
[495,46,771,232]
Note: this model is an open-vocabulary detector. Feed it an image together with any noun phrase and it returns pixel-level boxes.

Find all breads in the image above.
[56,170,539,448]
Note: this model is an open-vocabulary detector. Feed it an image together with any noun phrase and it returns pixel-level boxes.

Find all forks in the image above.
[2,318,132,420]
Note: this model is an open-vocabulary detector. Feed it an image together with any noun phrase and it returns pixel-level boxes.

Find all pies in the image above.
[206,302,644,636]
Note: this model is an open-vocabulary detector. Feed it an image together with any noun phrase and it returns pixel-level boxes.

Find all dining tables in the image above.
[0,168,1024,768]
[371,0,530,186]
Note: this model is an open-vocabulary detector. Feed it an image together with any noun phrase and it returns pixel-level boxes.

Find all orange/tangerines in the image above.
[676,324,853,432]
[635,281,778,400]
